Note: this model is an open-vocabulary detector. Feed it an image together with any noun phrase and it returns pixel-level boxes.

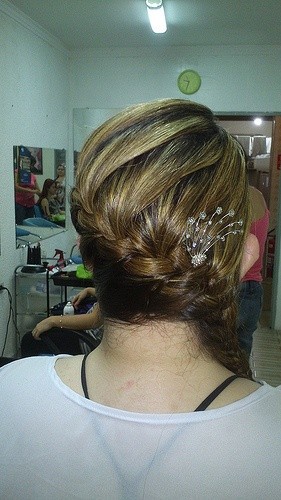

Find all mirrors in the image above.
[13,145,66,250]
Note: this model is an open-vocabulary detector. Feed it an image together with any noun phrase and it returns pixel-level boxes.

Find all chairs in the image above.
[33,203,42,218]
[23,218,62,228]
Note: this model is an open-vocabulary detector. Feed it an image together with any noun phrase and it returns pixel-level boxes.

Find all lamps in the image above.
[146,0,168,34]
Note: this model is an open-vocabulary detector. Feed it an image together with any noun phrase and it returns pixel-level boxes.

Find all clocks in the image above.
[178,70,201,94]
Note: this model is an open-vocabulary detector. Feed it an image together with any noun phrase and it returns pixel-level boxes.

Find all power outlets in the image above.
[0,282,4,294]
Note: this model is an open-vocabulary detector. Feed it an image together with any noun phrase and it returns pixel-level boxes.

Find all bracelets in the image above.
[60,315,64,329]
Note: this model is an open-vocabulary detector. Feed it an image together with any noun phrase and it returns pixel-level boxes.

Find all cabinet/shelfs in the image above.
[14,258,68,359]
[47,261,95,318]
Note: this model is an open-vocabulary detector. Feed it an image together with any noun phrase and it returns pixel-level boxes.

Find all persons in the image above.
[235,186,269,366]
[14,155,42,228]
[21,288,104,359]
[37,179,66,227]
[0,98,281,500]
[54,163,66,187]
